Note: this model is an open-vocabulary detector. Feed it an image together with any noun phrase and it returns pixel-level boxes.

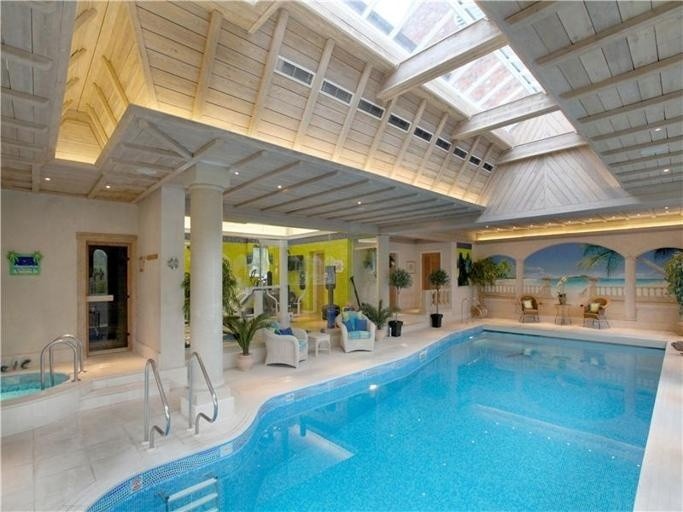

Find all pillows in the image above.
[348,317,367,331]
[274,327,292,335]
[523,299,533,308]
[590,302,600,313]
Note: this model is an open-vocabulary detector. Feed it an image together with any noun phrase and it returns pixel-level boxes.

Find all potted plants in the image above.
[388,267,411,336]
[221,312,273,370]
[427,266,450,327]
[470,255,512,318]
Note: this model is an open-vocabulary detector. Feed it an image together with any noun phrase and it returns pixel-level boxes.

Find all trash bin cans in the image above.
[327,309,336,329]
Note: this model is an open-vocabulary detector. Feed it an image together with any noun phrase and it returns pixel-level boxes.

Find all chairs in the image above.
[580,296,611,329]
[335,311,377,354]
[262,320,308,368]
[519,295,539,323]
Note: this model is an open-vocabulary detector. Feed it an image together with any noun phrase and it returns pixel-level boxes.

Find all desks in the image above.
[553,303,572,325]
[306,332,332,358]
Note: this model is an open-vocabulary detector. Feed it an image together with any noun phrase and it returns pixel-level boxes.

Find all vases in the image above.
[558,293,567,305]
